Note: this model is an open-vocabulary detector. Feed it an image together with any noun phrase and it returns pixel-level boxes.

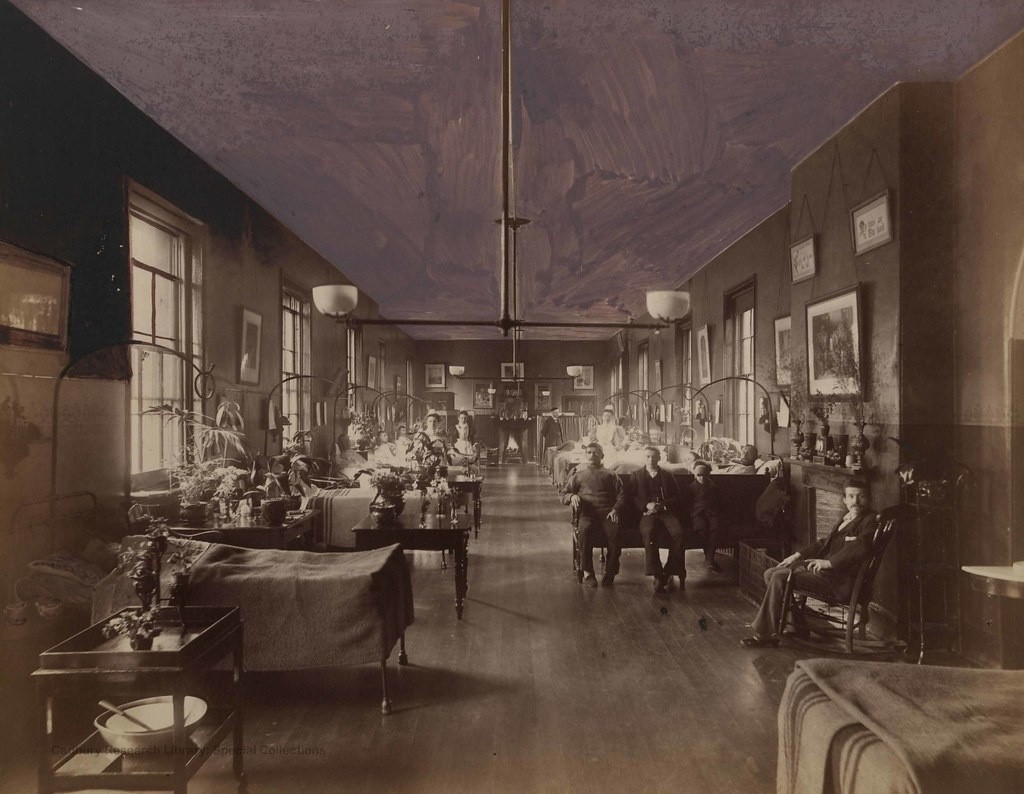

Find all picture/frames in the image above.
[366,354,377,390]
[534,382,553,411]
[771,186,896,402]
[325,367,348,399]
[694,323,713,389]
[653,358,663,396]
[424,363,447,389]
[472,379,495,409]
[396,375,402,401]
[0,240,73,355]
[575,365,594,390]
[500,362,525,382]
[615,332,624,352]
[236,303,264,388]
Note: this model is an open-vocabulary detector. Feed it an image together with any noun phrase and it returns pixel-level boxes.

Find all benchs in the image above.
[569,470,784,583]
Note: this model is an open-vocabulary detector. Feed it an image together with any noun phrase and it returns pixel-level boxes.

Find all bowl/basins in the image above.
[93,696,208,753]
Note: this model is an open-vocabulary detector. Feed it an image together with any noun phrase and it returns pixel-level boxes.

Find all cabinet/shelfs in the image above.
[28,604,246,794]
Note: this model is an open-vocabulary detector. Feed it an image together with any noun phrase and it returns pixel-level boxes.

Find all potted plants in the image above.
[368,471,402,527]
[266,442,330,510]
[166,438,206,522]
[99,517,198,652]
[148,401,243,510]
[214,464,244,517]
[245,453,300,525]
[378,463,407,516]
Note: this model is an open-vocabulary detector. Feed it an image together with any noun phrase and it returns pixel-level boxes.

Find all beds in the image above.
[26,434,1024,794]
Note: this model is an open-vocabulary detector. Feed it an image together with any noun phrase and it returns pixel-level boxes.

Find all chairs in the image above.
[771,503,904,656]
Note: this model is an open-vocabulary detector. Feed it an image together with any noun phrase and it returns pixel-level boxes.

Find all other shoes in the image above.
[740,635,780,647]
[602,573,615,585]
[584,574,598,587]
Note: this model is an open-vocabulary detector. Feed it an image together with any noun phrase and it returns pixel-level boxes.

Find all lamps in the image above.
[447,215,582,380]
[308,0,692,328]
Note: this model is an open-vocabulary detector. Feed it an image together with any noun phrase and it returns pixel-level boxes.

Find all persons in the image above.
[589,406,622,460]
[624,446,686,592]
[377,411,477,475]
[687,450,699,464]
[740,480,882,649]
[686,461,722,569]
[542,408,563,449]
[727,444,757,474]
[559,443,624,587]
